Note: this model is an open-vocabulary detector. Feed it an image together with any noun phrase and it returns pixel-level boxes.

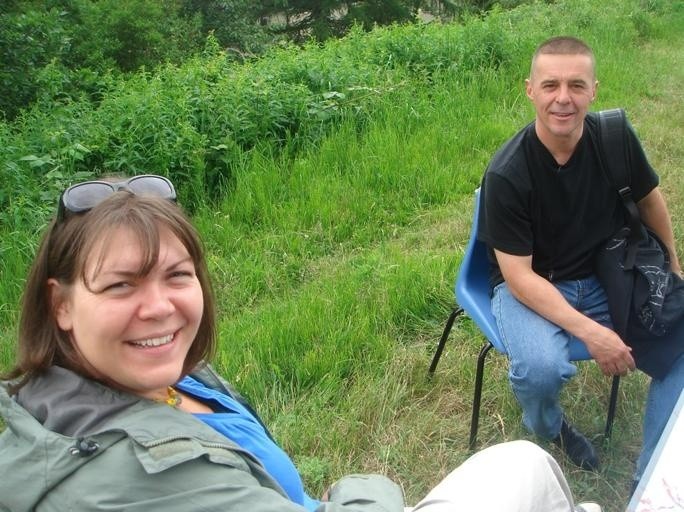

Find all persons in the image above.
[481,34,684,503]
[0,172,608,512]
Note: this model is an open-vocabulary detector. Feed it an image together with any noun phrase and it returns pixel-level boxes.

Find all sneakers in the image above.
[573,500,602,512]
[556,418,600,469]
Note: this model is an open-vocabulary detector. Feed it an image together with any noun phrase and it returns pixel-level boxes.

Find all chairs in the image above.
[429,185,620,456]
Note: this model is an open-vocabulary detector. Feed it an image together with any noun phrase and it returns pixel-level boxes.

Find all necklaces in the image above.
[149,384,186,409]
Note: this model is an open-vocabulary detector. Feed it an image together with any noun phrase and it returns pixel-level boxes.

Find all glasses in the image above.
[55,175,179,224]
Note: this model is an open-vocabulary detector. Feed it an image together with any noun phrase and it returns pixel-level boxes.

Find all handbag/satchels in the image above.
[596,220,666,286]
